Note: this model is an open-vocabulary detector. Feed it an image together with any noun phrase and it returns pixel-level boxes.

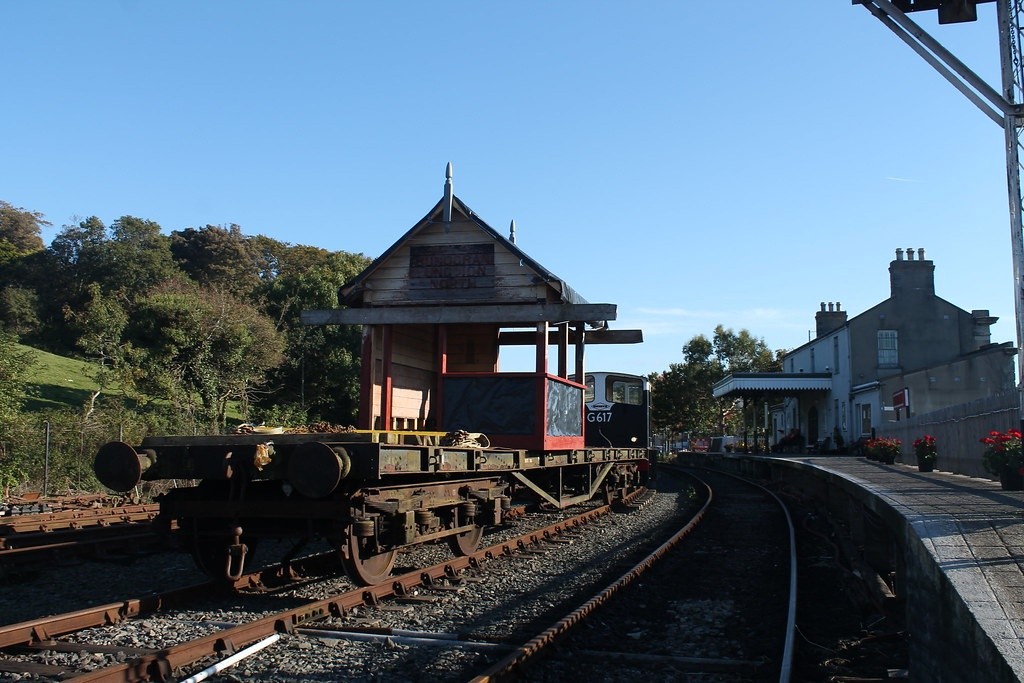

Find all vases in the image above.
[919,460,933,472]
[1000,475,1024,490]
[867,456,894,465]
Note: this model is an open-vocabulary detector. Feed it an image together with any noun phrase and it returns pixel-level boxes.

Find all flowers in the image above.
[864,436,903,457]
[978,428,1024,476]
[912,434,938,460]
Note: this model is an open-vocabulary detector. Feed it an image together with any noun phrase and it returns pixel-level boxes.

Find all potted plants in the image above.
[724,440,767,455]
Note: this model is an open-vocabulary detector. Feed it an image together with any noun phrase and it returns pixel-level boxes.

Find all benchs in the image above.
[805,437,829,454]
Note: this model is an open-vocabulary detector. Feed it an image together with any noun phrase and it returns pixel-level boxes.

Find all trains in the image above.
[91,371,660,597]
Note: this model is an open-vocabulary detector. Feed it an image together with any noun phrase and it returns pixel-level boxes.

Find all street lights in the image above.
[42,419,49,496]
